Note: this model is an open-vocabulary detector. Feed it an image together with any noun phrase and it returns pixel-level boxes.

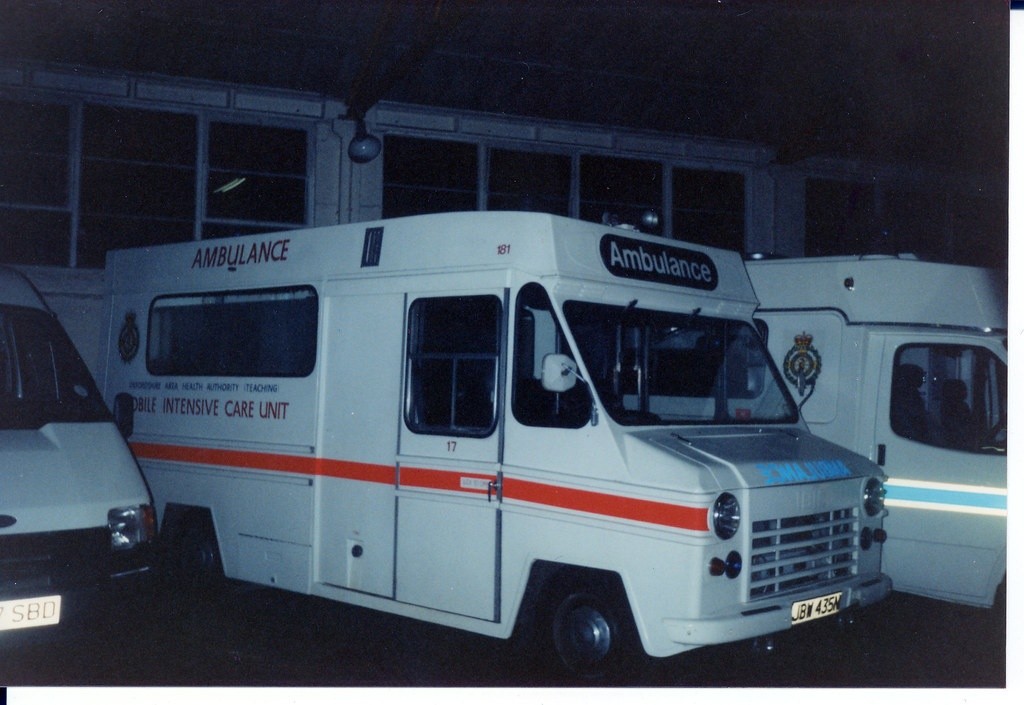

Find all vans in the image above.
[0,261,160,645]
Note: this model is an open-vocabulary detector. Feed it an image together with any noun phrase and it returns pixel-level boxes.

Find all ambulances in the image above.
[617,248,1007,618]
[95,211,890,689]
